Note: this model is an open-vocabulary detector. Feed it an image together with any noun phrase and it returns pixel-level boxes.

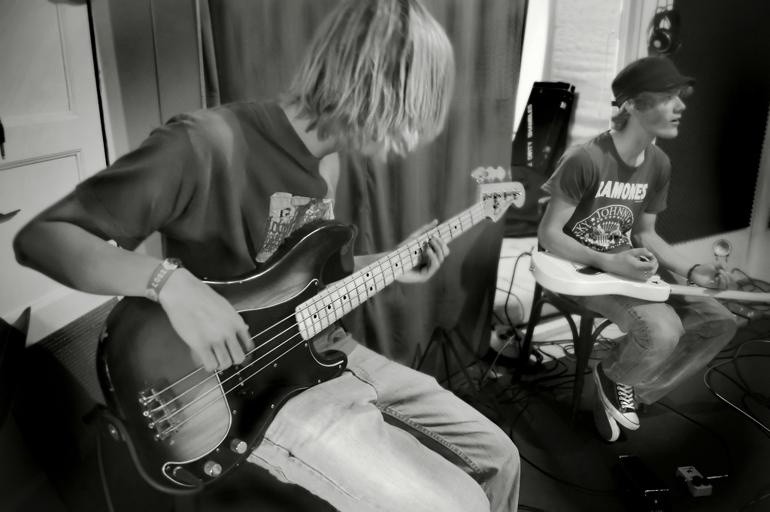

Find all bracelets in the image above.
[144,257,183,303]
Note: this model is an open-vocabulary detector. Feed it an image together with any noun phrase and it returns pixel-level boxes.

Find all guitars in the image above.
[95,166,526,496]
[529,250,770,303]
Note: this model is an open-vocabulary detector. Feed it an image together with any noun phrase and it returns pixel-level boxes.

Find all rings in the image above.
[644,272,647,277]
[687,264,700,285]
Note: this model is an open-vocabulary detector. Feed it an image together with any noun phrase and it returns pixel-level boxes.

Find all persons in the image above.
[12,0,521,512]
[537,58,738,442]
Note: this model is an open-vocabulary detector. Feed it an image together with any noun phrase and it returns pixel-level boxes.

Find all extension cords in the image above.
[678,465,714,499]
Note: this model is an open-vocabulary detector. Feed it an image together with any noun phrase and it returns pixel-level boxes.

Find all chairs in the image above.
[506,191,622,439]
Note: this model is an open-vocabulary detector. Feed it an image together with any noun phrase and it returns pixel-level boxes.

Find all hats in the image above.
[612,56,695,107]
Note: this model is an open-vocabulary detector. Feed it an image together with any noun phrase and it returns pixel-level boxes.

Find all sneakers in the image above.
[592,362,641,442]
